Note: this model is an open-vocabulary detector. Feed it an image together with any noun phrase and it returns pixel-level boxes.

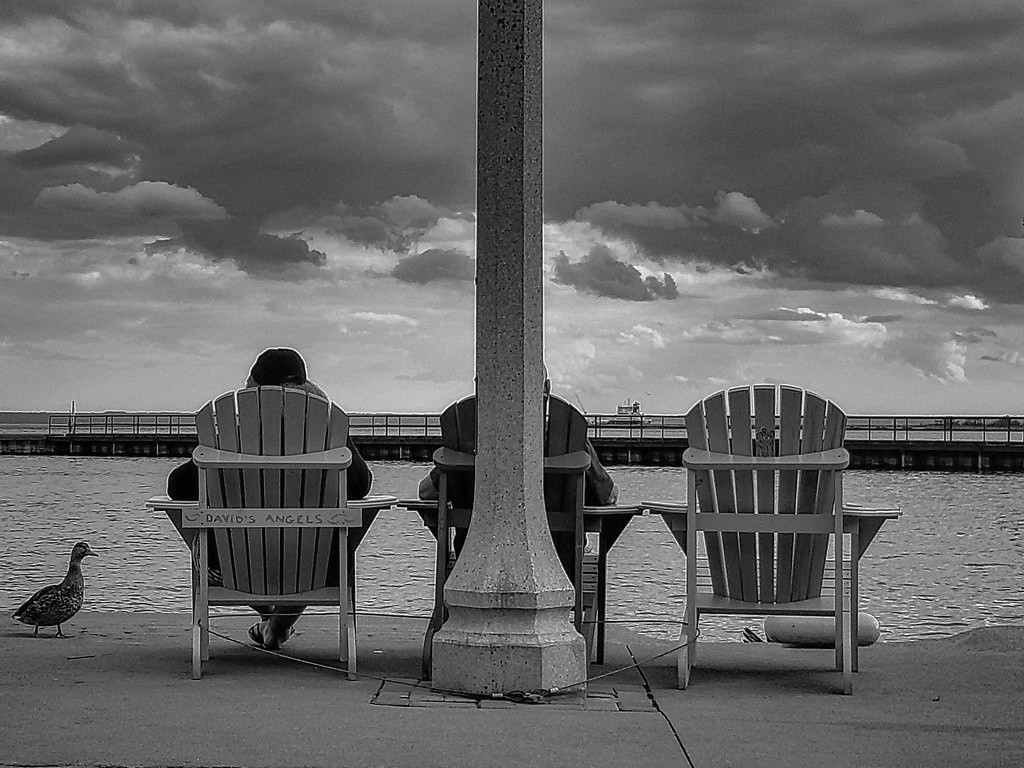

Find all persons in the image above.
[168,349,372,651]
[419,363,619,586]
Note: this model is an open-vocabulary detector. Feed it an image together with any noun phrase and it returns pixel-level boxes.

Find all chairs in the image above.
[644,382,902,693]
[144,386,397,683]
[396,392,643,679]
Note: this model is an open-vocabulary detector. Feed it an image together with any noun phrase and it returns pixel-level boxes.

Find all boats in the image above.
[884,425,902,429]
[912,420,944,430]
[606,397,652,425]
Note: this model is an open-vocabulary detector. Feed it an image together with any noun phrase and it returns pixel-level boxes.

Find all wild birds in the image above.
[11,541,100,638]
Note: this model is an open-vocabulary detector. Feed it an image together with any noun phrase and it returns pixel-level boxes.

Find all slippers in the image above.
[247,622,296,651]
